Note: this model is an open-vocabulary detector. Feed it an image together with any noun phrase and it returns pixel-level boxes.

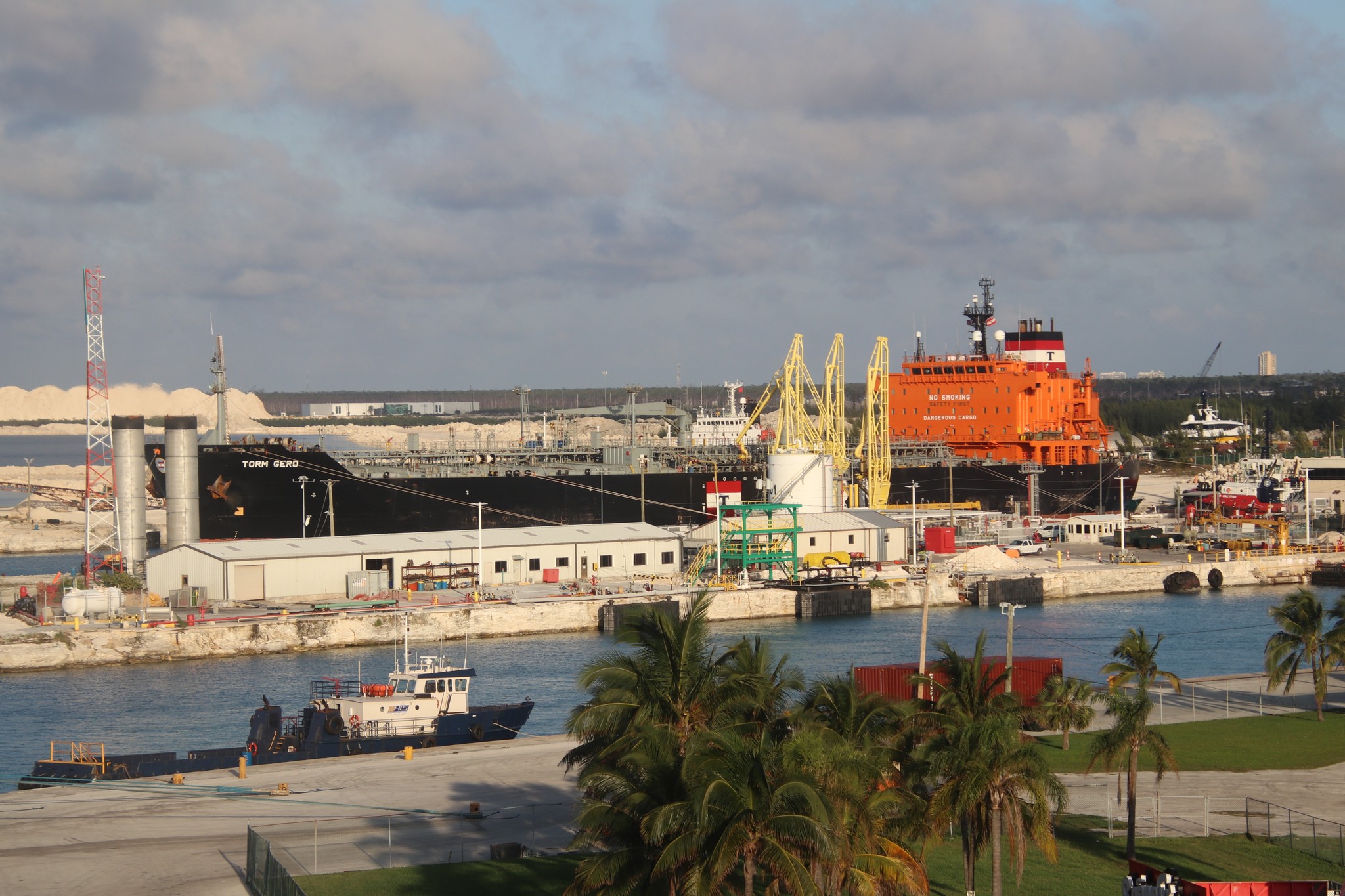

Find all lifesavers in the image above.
[1166,576,1175,586]
[324,715,344,735]
[249,742,257,757]
[432,696,439,708]
[351,715,359,728]
[1187,571,1195,576]
[349,749,365,755]
[1170,572,1180,579]
[1175,576,1185,585]
[1227,488,1231,493]
[1181,571,1190,577]
[422,736,437,748]
[471,724,485,740]
[942,461,947,466]
[677,467,682,473]
[1208,568,1223,588]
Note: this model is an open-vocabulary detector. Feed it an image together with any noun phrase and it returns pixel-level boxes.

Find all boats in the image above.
[1179,459,1313,527]
[1157,389,1255,455]
[11,594,538,791]
[850,276,1141,519]
[686,379,776,448]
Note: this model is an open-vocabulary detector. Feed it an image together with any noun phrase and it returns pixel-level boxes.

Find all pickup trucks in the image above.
[1002,539,1047,555]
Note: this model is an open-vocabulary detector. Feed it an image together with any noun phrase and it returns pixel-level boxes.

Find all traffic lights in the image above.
[1191,465,1217,519]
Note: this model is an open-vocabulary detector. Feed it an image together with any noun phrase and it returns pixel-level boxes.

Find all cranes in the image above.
[733,332,894,512]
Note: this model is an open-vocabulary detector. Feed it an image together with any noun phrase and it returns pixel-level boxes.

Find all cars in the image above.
[1033,524,1065,542]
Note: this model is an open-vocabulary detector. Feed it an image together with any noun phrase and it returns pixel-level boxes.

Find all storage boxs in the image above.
[853,657,1063,709]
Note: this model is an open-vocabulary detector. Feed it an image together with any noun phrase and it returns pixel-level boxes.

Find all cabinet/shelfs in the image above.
[401,562,480,592]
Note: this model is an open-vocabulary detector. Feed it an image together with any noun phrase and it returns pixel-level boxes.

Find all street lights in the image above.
[1000,601,1028,697]
[292,475,315,538]
[904,481,921,568]
[1114,476,1130,555]
[511,385,531,446]
[913,551,935,700]
[1090,447,1109,516]
[1298,467,1315,546]
[470,502,489,591]
[624,383,642,448]
[1333,421,1340,456]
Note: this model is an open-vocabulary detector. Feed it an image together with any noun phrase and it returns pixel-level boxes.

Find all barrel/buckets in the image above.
[565,470,569,474]
[558,441,562,448]
[307,447,314,452]
[850,552,865,558]
[751,563,755,569]
[292,447,295,451]
[876,563,882,571]
[526,441,537,448]
[427,586,427,587]
[434,579,441,590]
[488,471,498,476]
[907,555,913,563]
[441,579,447,589]
[241,751,252,766]
[35,525,38,530]
[146,531,160,550]
[558,470,561,475]
[71,567,76,577]
[20,586,27,598]
[360,473,364,477]
[772,565,776,570]
[684,466,692,473]
[1262,542,1268,551]
[737,460,741,464]
[368,473,371,477]
[300,446,303,452]
[417,581,424,591]
